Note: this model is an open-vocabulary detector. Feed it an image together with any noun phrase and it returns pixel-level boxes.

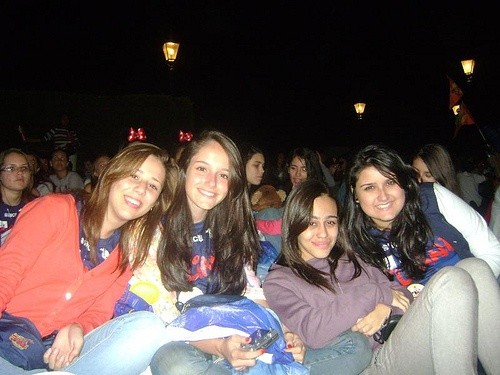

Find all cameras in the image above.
[239,328,279,352]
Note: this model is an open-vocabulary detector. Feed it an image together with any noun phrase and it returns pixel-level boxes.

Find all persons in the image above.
[127,132,306,375]
[342,148,500,276]
[0,143,179,375]
[412,143,499,241]
[242,144,344,288]
[263,178,500,375]
[0,148,112,241]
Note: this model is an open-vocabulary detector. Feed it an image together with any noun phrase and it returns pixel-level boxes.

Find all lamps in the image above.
[461,56,475,82]
[162,31,180,64]
[353,97,366,120]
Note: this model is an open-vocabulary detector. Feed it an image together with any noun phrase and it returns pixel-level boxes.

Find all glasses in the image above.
[1,165,32,175]
